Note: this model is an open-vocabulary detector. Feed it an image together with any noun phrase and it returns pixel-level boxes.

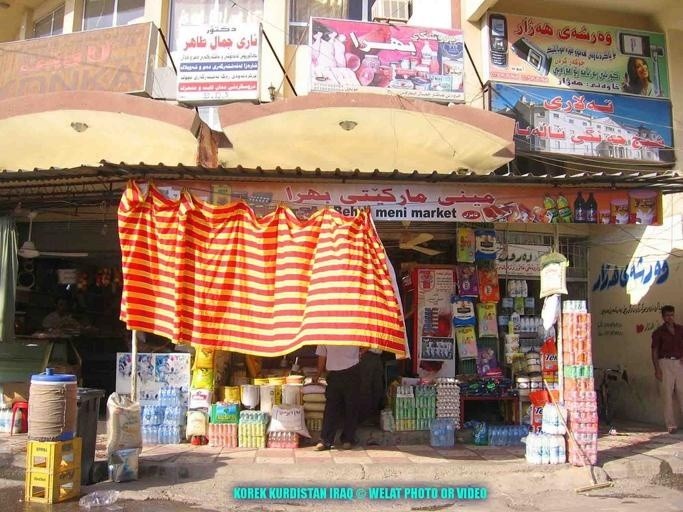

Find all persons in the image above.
[361,351,385,427]
[44,296,79,364]
[313,344,361,450]
[622,58,656,96]
[651,306,683,435]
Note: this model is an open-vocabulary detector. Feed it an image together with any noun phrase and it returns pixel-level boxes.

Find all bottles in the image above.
[520,317,543,332]
[141,385,185,444]
[555,192,573,223]
[586,192,597,223]
[421,38,440,75]
[428,417,455,446]
[562,300,586,313]
[543,192,562,223]
[267,431,300,449]
[507,280,528,298]
[519,339,540,353]
[489,424,529,446]
[525,401,569,463]
[574,191,586,223]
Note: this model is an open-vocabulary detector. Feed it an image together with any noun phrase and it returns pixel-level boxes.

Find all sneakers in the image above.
[313,441,330,451]
[341,443,353,451]
[667,428,676,435]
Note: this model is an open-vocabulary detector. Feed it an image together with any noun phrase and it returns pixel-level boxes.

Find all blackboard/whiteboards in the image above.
[419,336,455,362]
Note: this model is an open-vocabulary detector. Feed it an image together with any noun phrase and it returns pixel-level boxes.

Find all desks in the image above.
[458,394,519,431]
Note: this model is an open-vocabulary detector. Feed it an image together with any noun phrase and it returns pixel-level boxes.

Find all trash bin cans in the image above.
[75,388,105,487]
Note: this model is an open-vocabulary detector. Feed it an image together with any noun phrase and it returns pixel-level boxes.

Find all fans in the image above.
[15,210,90,258]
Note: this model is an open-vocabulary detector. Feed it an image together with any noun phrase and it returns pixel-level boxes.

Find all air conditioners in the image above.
[369,0,409,26]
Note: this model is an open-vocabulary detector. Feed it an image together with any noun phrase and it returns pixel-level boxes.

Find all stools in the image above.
[8,402,27,435]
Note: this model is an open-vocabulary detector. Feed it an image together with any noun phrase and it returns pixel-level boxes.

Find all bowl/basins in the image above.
[285,376,304,383]
[268,377,285,385]
[248,378,269,384]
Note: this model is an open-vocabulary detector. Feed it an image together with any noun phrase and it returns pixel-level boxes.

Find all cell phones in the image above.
[619,32,651,58]
[489,14,508,66]
[517,39,553,77]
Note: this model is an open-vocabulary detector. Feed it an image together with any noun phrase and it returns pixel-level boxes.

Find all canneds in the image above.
[209,410,269,448]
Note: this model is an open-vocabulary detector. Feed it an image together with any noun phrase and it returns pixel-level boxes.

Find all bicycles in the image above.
[593,363,621,425]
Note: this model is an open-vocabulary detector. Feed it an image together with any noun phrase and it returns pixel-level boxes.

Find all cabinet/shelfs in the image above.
[14,285,105,333]
